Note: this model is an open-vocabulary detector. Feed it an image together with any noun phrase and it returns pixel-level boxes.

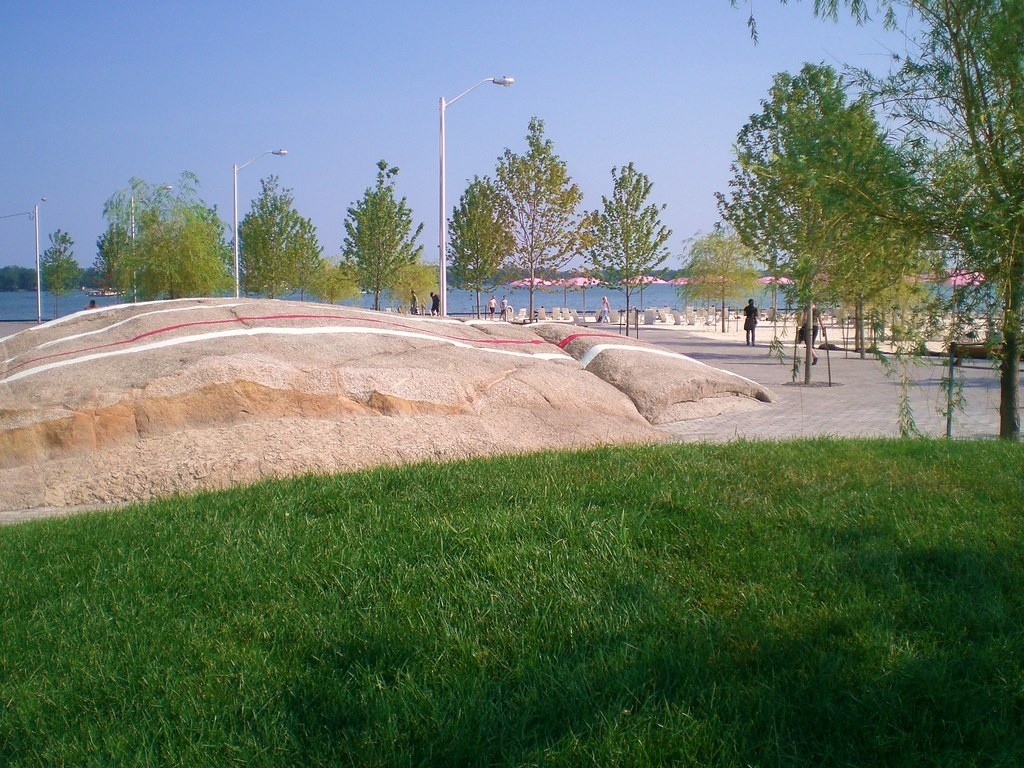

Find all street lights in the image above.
[131,185,173,303]
[234,149,288,297]
[35,198,47,323]
[439,75,515,318]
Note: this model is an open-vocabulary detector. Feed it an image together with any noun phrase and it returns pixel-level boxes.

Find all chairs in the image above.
[508,305,730,326]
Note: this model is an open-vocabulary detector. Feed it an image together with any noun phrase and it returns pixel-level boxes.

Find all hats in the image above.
[430,292,433,297]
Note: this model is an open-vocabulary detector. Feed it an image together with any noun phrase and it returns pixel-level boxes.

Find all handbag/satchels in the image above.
[798,326,806,342]
[597,312,603,322]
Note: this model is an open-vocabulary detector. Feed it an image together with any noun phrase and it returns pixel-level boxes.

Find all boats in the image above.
[84,288,126,297]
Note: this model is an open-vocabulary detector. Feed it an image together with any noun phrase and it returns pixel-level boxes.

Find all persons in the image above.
[397,305,405,313]
[370,304,375,310]
[85,300,97,310]
[802,301,827,365]
[410,289,418,314]
[429,292,440,317]
[499,296,508,320]
[744,299,758,346]
[487,295,500,321]
[599,296,610,323]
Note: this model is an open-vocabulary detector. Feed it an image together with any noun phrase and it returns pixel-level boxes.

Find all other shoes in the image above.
[804,361,806,364]
[812,357,818,365]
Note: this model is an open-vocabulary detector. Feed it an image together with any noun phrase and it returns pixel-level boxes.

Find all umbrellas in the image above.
[510,277,613,322]
[689,273,735,307]
[909,268,986,287]
[615,275,668,311]
[667,277,692,322]
[755,277,794,308]
